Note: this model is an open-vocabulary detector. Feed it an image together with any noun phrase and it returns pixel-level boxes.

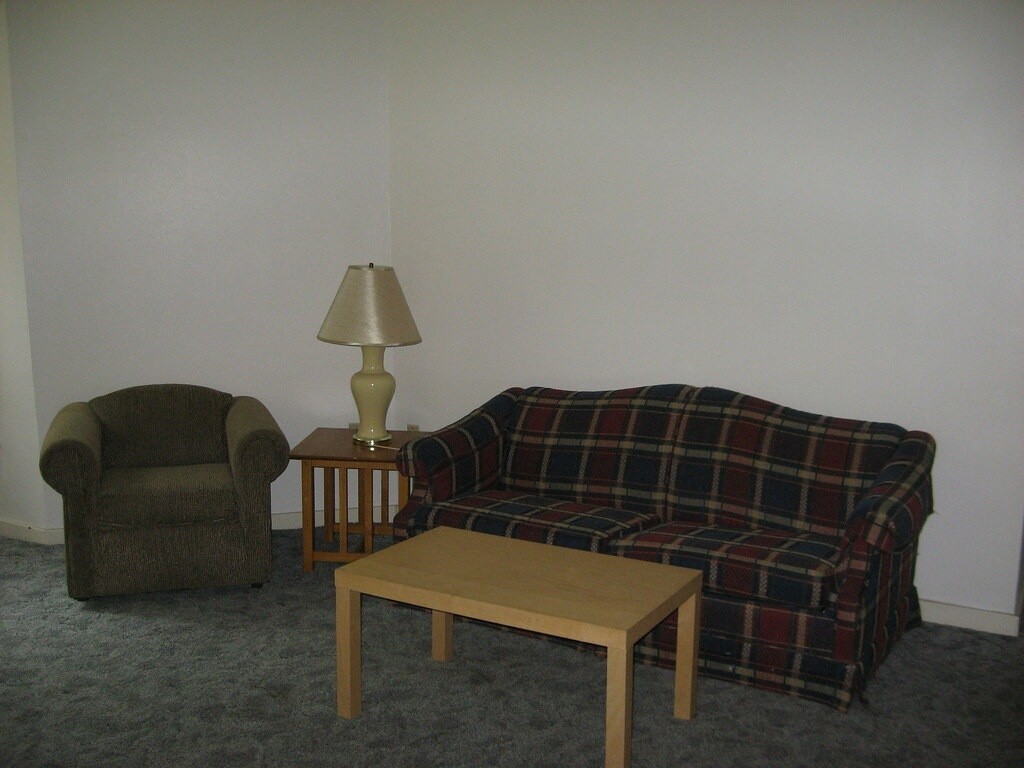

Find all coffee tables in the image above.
[336,524,703,768]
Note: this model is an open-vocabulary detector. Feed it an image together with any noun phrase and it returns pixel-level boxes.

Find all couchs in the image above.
[38,383,293,601]
[404,366,939,693]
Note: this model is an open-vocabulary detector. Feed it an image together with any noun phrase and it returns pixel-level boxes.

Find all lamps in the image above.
[316,265,414,437]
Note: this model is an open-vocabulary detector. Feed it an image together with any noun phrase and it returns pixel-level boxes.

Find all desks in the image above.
[291,428,419,563]
